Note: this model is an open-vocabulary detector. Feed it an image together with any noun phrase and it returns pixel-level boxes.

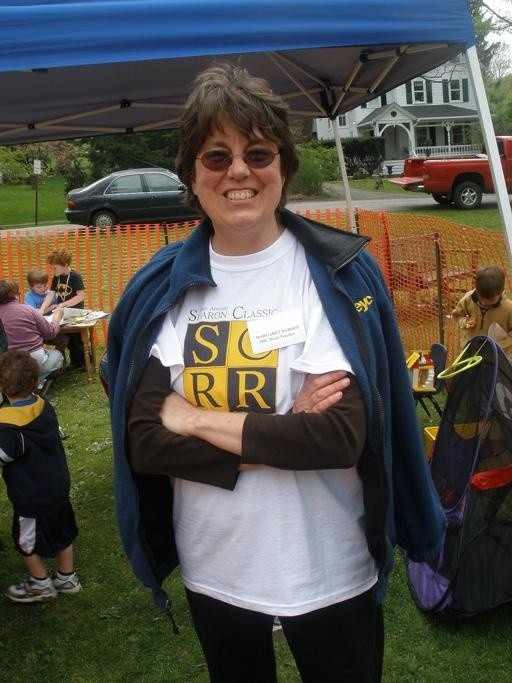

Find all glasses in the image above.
[196,148,280,171]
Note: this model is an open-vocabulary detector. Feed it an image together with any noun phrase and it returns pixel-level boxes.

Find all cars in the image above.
[62,164,202,232]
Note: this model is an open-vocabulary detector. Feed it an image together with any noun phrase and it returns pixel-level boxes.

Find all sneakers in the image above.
[3,574,59,604]
[49,569,83,595]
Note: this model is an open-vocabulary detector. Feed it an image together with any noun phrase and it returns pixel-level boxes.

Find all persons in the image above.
[1,279,64,389]
[99,61,447,682]
[1,351,83,605]
[452,266,512,361]
[23,271,69,365]
[47,251,85,372]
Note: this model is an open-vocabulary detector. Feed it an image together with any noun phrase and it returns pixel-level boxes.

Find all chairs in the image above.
[375,231,485,327]
[0,319,56,400]
[408,342,448,419]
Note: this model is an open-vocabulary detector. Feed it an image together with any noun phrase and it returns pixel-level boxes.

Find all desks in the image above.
[50,310,99,384]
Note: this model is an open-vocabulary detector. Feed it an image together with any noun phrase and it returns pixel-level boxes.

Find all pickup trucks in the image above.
[386,133,512,210]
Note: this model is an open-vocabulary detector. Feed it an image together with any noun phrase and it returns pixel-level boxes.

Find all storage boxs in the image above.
[423,420,507,462]
